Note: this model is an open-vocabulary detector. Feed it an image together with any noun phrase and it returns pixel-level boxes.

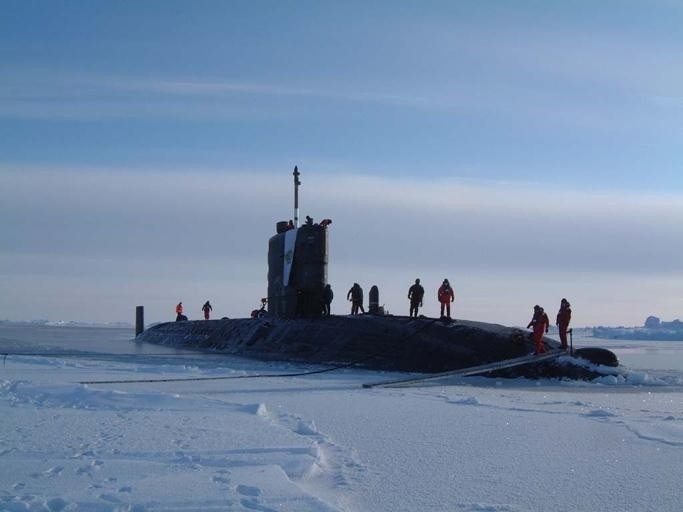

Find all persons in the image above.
[527,303,550,357]
[556,297,572,350]
[287,220,293,229]
[318,219,331,228]
[302,215,312,227]
[174,277,454,323]
